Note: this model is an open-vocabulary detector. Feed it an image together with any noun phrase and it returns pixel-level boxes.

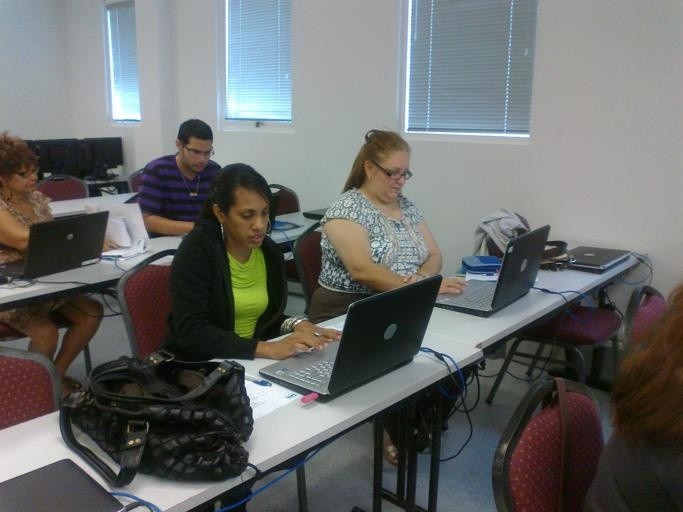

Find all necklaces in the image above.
[174,158,201,197]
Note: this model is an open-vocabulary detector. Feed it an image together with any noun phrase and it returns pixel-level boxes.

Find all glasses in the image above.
[13,166,40,179]
[179,142,214,159]
[369,160,412,180]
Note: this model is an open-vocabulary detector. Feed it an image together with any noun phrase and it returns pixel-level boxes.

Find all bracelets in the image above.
[416,272,429,279]
[277,314,310,333]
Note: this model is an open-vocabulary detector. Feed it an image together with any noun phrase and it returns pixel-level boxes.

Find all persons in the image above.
[575,285,682,512]
[133,117,223,237]
[160,163,343,512]
[304,130,471,470]
[0,131,120,402]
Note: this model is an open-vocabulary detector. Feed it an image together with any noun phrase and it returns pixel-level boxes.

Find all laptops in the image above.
[0,459,127,512]
[567,246,631,274]
[259,275,443,403]
[434,225,550,317]
[0,211,109,280]
[303,208,328,220]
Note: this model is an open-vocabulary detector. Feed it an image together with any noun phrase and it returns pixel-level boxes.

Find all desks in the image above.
[348,245,643,509]
[1,320,484,511]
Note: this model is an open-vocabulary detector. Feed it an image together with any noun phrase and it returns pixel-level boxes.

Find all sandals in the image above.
[383,443,398,465]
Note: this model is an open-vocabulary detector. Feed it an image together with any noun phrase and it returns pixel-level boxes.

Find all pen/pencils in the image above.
[245,375,272,386]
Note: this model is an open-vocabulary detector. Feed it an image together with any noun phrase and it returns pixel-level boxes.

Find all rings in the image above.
[312,331,325,338]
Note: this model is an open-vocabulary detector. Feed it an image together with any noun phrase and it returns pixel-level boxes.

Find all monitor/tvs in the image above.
[23,136,123,181]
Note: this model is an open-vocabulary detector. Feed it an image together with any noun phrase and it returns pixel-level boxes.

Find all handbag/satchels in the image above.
[58,348,253,487]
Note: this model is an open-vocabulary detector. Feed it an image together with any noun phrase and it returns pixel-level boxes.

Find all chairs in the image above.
[491,370,613,509]
[623,280,670,348]
[296,218,332,325]
[1,344,62,432]
[480,216,621,408]
[1,171,315,373]
[115,246,310,512]
